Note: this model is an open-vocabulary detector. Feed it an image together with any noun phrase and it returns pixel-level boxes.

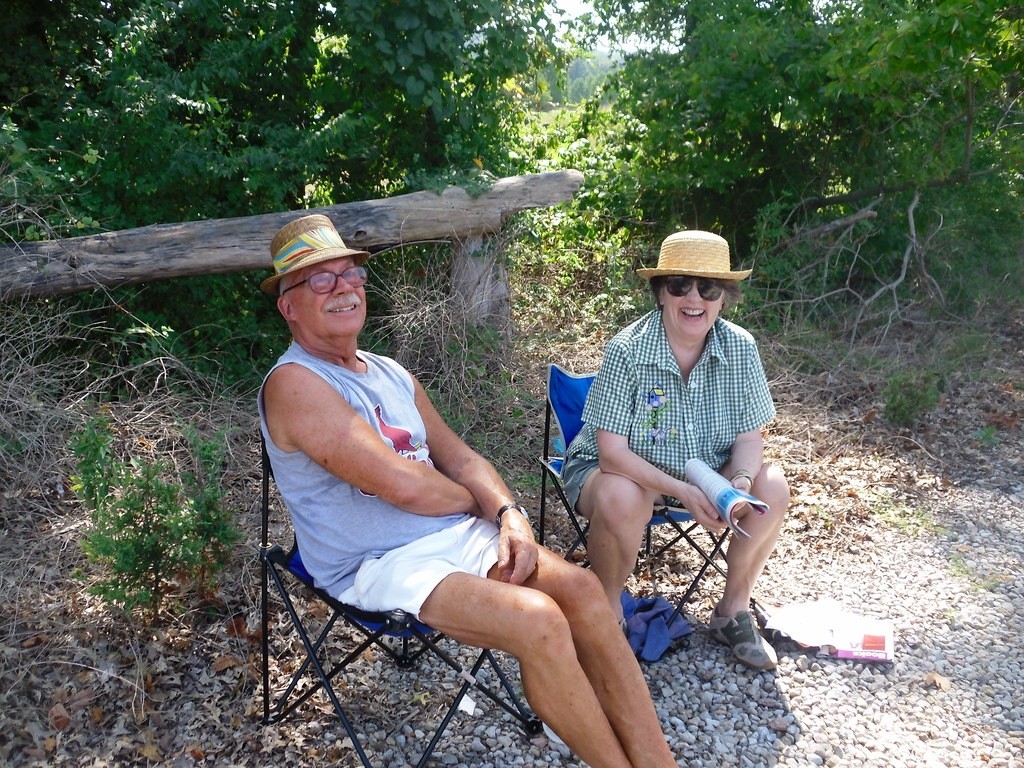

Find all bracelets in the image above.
[495,503,530,531]
[729,468,755,491]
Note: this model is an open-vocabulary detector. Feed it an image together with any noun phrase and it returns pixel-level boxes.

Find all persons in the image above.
[559,230,790,670]
[257,214,678,768]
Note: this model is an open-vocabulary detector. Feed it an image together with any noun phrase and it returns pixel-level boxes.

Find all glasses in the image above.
[662,275,726,301]
[282,266,367,295]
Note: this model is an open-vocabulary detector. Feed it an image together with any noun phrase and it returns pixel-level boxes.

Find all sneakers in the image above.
[710,601,778,670]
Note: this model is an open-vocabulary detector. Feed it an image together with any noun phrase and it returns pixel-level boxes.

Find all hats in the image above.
[636,231,753,281]
[260,214,372,294]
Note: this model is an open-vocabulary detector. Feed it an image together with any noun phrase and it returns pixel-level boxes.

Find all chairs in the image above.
[539,363,782,641]
[256,428,543,768]
